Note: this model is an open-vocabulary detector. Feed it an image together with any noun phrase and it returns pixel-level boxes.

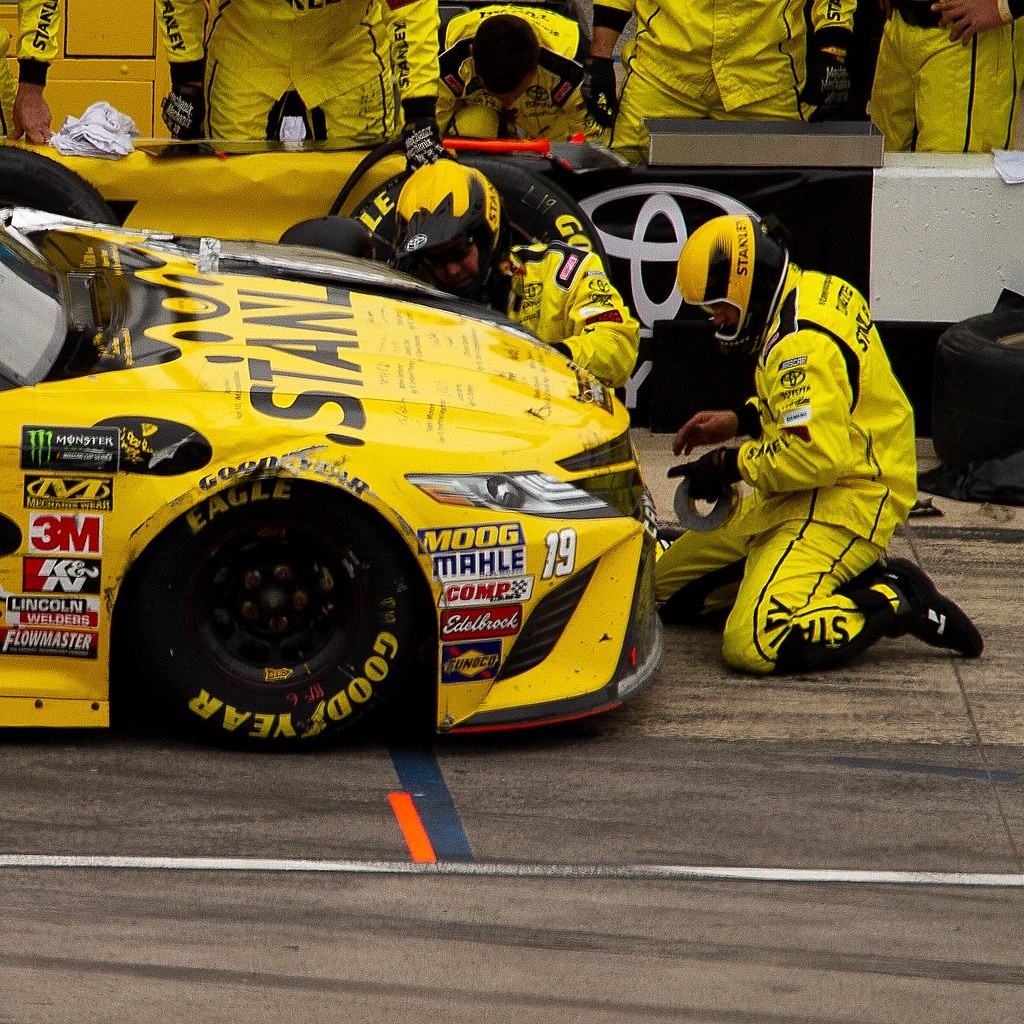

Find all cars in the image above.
[2,197,659,750]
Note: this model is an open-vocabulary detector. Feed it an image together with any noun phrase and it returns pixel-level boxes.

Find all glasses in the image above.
[423,236,474,274]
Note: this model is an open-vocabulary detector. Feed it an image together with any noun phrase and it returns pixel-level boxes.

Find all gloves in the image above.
[581,54,620,129]
[800,43,853,123]
[160,83,207,140]
[665,446,734,505]
[402,120,455,181]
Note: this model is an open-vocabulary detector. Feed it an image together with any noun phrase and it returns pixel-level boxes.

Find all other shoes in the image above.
[885,556,984,657]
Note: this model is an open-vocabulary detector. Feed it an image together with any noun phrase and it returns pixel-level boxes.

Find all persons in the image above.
[0,0,62,150]
[389,158,642,390]
[278,215,394,269]
[653,213,984,677]
[152,0,458,182]
[395,0,611,145]
[581,0,861,149]
[865,0,1024,154]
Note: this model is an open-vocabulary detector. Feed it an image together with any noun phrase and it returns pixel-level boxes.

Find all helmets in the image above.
[676,214,790,362]
[392,158,500,257]
